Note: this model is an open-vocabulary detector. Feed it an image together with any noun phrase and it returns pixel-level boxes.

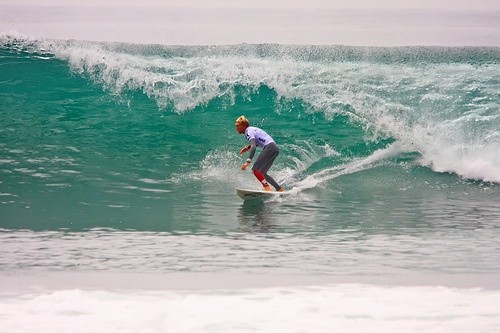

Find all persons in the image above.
[235,116,284,194]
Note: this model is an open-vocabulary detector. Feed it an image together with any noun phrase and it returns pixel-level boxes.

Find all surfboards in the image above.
[236,188,284,198]
